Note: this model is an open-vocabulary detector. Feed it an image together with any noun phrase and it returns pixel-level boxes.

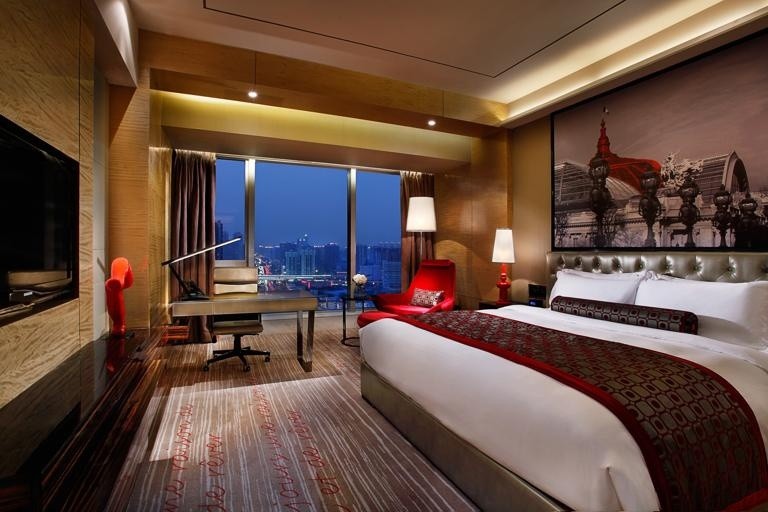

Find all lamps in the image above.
[161,236,243,300]
[405,196,437,262]
[490,227,516,305]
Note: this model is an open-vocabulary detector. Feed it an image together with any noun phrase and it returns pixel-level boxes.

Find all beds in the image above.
[358,247,767,512]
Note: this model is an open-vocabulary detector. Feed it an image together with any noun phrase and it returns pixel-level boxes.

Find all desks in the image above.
[171,291,320,372]
[339,294,371,347]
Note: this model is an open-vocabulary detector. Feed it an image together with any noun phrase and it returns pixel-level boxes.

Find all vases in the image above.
[354,285,368,298]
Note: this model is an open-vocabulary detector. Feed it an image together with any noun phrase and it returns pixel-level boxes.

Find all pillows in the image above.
[548,267,767,350]
[409,287,445,308]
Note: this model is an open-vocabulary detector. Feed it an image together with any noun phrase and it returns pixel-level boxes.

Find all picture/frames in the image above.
[550,28,767,254]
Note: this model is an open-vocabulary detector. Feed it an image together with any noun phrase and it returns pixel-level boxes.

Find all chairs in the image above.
[201,267,271,373]
[370,259,457,317]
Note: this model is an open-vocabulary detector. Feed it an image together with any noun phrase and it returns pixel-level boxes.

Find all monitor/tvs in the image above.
[0,114,79,327]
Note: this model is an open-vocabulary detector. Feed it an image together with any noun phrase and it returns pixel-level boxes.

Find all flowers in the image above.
[352,273,368,289]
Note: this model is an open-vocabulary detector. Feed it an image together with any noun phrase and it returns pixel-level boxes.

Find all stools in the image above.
[357,312,398,329]
